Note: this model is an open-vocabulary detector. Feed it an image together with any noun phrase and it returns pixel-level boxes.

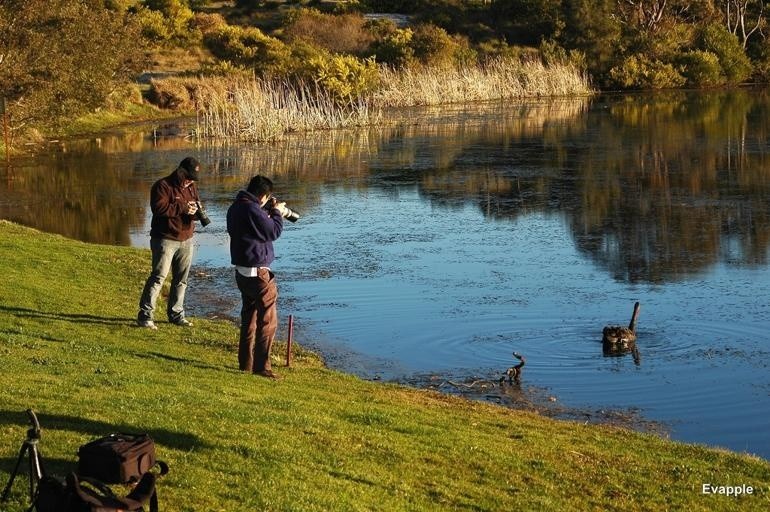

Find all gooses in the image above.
[602,301,640,343]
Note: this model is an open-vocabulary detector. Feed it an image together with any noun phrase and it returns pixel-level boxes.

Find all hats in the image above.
[180,157,202,182]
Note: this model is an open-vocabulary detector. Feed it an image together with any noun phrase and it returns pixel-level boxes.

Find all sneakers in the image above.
[170,318,195,327]
[136,320,159,330]
[253,369,286,381]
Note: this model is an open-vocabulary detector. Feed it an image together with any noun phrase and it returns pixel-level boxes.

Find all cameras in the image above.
[191,200,211,228]
[265,196,301,223]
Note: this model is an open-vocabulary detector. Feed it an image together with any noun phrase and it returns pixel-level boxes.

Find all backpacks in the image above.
[35,470,160,512]
[75,430,170,486]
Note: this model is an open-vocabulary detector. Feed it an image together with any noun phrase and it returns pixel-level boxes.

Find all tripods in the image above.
[0,444,45,506]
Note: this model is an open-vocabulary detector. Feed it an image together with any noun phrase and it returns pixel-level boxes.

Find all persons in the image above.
[135,156,208,330]
[226,172,287,377]
